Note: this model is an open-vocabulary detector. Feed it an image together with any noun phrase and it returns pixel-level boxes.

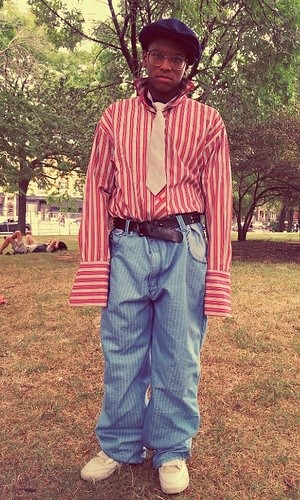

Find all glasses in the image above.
[146,50,189,71]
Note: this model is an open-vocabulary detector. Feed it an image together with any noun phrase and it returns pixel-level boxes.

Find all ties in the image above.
[145,90,178,197]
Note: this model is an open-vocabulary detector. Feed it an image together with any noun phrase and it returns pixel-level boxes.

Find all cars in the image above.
[260,221,300,232]
[0,218,32,236]
[234,222,253,233]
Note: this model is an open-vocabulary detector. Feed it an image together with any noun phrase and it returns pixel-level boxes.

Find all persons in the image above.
[249,222,253,228]
[0,230,68,254]
[59,213,65,227]
[295,223,299,232]
[68,19,233,495]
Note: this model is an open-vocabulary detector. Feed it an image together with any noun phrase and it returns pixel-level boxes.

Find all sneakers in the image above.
[159,459,189,494]
[81,450,123,483]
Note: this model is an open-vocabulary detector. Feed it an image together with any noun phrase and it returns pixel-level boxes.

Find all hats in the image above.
[139,18,201,62]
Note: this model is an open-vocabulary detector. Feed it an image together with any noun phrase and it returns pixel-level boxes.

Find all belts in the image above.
[113,213,199,243]
